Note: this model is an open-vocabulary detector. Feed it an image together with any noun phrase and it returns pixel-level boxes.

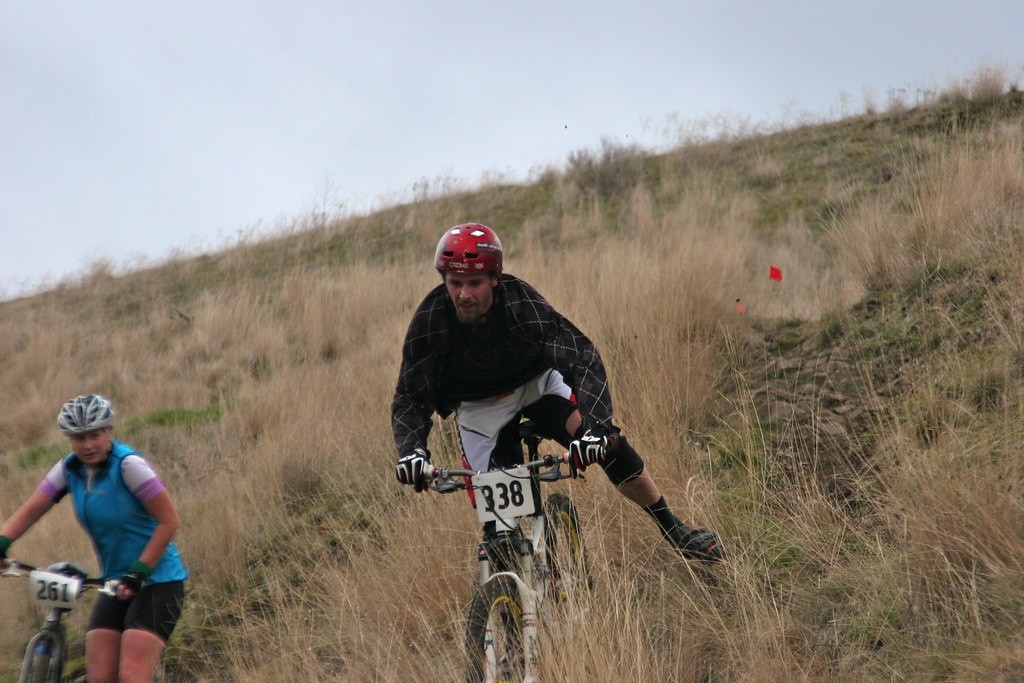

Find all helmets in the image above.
[434,222,503,279]
[57,395,114,436]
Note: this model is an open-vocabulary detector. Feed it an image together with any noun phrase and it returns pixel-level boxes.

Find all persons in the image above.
[391,222,725,683]
[0,392,190,683]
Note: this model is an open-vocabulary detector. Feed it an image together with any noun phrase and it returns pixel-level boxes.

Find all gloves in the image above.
[396,448,430,493]
[569,427,608,479]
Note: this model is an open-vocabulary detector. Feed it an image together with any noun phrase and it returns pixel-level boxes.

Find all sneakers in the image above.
[671,528,724,563]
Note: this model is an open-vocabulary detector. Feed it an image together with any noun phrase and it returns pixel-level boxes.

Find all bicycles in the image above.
[0,557,137,683]
[424,431,609,683]
